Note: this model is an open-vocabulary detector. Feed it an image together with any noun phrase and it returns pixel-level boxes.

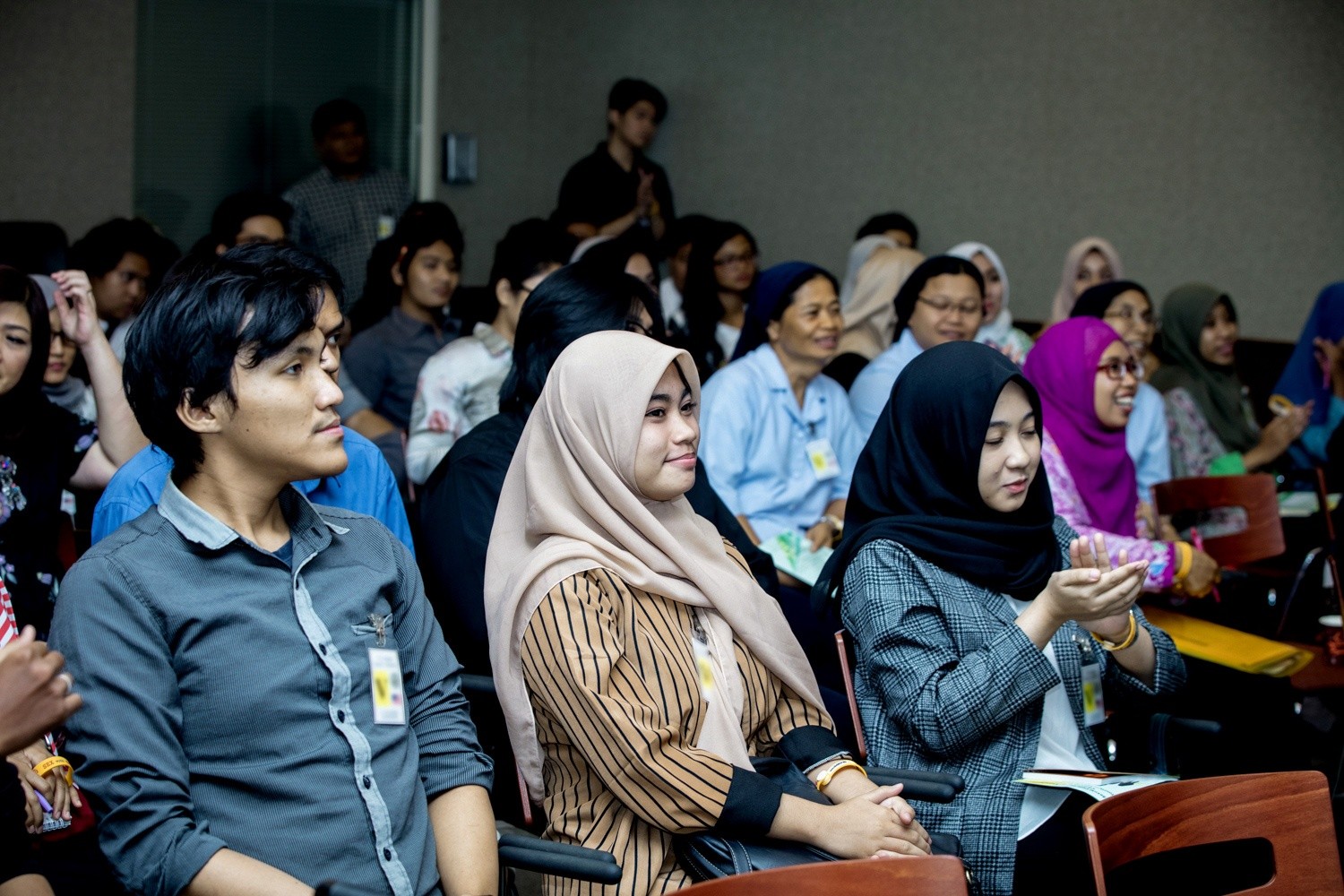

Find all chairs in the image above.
[453,624,1344,896]
[1148,472,1287,571]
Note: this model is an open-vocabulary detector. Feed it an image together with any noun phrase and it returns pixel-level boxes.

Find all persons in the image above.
[0,209,1344,896]
[283,103,413,314]
[837,340,1344,896]
[0,623,85,896]
[483,325,931,896]
[49,238,499,896]
[557,75,678,237]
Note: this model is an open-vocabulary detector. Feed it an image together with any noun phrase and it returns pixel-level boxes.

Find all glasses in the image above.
[918,294,982,316]
[712,248,762,267]
[1095,360,1148,381]
[50,327,79,348]
[1104,307,1154,325]
[118,270,150,290]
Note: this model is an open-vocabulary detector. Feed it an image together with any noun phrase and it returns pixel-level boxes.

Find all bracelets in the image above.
[33,755,74,788]
[1173,539,1193,579]
[820,513,844,534]
[815,759,868,792]
[1091,608,1138,652]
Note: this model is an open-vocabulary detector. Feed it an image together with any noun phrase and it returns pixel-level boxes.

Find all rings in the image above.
[57,674,73,696]
